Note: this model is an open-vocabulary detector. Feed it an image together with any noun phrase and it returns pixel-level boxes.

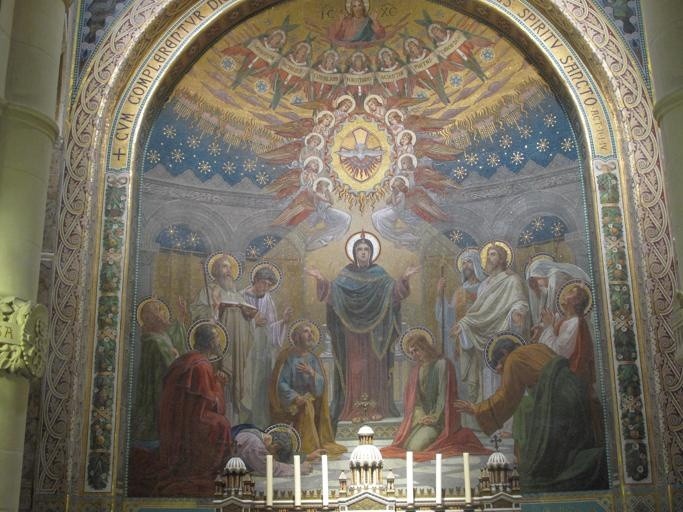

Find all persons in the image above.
[303,239,423,423]
[189,254,258,430]
[450,240,529,439]
[238,265,291,434]
[526,260,594,341]
[266,319,344,459]
[450,337,609,493]
[135,290,186,444]
[433,250,488,330]
[391,334,461,454]
[538,286,596,389]
[159,321,228,483]
[227,423,325,477]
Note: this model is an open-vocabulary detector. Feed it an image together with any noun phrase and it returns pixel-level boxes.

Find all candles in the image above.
[436,453,442,504]
[293,455,302,507]
[267,455,273,506]
[463,453,471,503]
[322,454,328,506]
[406,451,414,504]
